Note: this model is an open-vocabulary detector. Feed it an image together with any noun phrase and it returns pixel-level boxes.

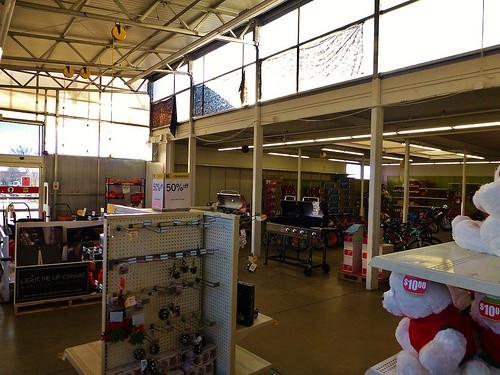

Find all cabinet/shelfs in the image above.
[392,185,448,209]
[364,241,500,375]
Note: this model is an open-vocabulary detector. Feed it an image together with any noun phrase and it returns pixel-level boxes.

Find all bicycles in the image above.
[262,205,454,252]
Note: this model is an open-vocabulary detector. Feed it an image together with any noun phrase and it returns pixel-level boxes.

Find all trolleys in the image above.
[264,200,332,278]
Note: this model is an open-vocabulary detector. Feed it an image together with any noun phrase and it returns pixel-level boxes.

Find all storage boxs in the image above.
[342,225,394,279]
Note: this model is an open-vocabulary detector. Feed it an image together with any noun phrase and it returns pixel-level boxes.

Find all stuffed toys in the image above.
[382,271,500,375]
[451,164,500,256]
